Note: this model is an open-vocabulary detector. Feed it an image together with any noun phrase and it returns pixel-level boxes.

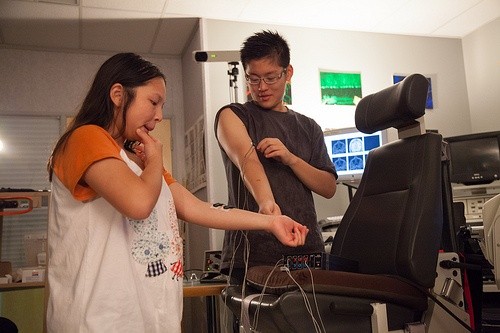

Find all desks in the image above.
[183,283,230,333]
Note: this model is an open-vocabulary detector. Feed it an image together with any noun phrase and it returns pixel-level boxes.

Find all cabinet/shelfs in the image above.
[0,282,45,333]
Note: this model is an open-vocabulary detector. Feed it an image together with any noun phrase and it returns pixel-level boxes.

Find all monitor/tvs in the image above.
[323,127,387,181]
[444,131,500,185]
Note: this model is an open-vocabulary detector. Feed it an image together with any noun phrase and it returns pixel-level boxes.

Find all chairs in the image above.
[221,73,443,333]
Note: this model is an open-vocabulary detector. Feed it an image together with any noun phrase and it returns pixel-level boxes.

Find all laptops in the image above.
[200,250,228,282]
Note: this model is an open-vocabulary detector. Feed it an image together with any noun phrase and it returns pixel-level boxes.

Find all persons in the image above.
[214,30,338,333]
[45,52,309,333]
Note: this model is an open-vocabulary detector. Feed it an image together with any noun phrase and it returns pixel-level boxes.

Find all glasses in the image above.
[245,69,285,86]
